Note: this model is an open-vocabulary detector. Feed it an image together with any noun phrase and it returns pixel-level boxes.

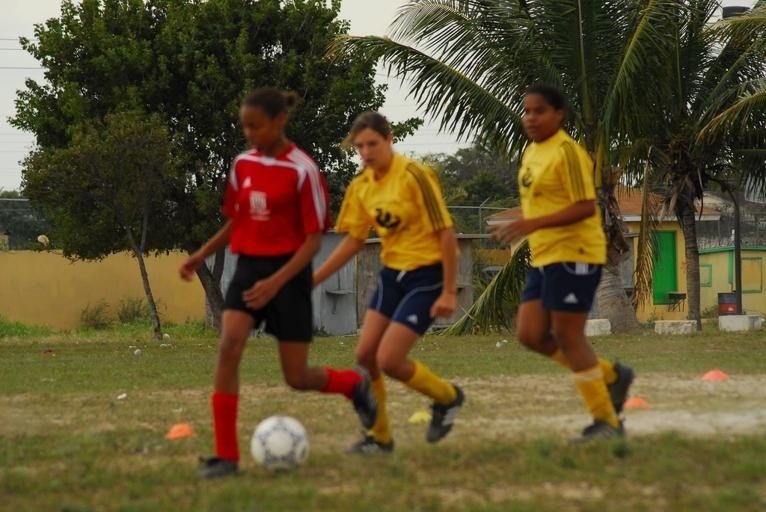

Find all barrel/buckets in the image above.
[717,291,739,316]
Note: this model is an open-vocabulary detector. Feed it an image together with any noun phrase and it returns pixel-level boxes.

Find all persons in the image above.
[309,112,465,453]
[178,88,376,478]
[484,84,634,442]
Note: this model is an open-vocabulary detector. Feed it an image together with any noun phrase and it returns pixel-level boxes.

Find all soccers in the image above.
[251,415,310,474]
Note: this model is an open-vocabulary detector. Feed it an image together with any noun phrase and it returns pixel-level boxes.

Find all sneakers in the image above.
[346,370,395,458]
[196,458,238,481]
[428,384,464,442]
[569,364,641,447]
